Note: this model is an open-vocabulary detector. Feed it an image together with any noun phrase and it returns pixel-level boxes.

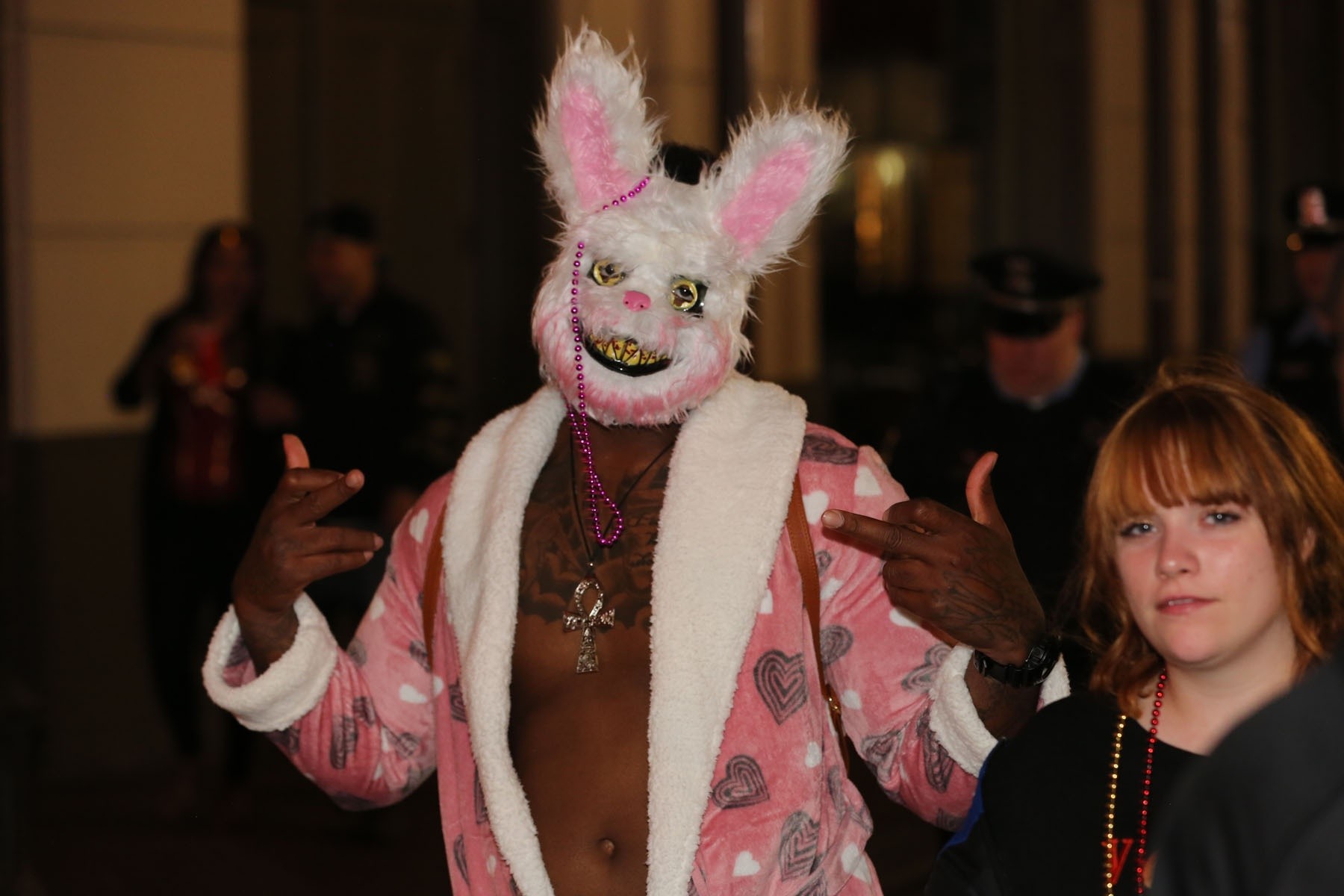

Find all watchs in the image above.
[973,625,1064,688]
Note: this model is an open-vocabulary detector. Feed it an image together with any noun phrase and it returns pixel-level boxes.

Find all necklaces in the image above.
[561,410,677,676]
[1105,710,1125,896]
[567,171,650,545]
[1135,667,1167,896]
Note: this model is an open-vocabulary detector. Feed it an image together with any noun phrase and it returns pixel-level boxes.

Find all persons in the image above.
[910,355,1344,896]
[199,26,1076,895]
[1267,174,1344,479]
[891,248,1162,630]
[108,211,428,784]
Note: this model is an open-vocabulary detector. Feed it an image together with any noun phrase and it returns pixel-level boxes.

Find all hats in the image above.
[1278,182,1344,250]
[969,248,1102,337]
